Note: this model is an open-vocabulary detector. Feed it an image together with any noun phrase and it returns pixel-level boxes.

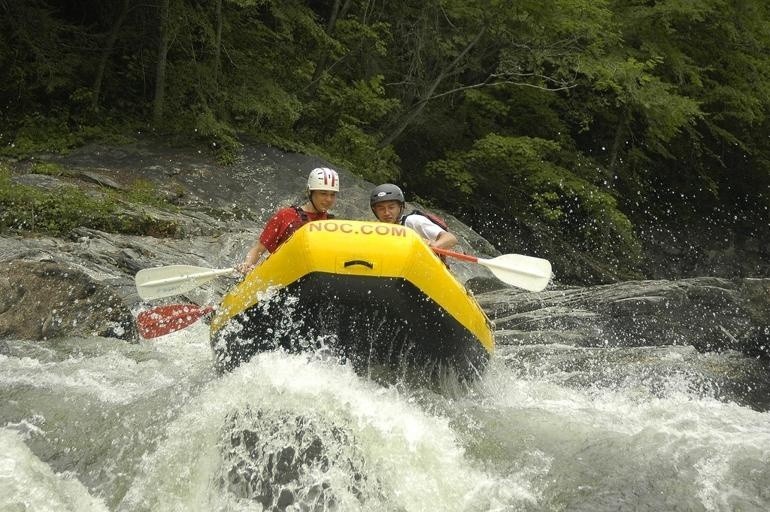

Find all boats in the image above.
[208,217,498,403]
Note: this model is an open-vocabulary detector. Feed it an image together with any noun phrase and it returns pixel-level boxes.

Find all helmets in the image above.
[305,168,340,193]
[368,182,404,206]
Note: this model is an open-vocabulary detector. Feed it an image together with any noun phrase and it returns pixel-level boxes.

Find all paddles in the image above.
[136,303,215,339]
[428,242,552,292]
[135,264,237,299]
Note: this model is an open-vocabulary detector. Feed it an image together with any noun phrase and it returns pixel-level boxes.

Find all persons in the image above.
[232,166,340,278]
[369,185,458,272]
